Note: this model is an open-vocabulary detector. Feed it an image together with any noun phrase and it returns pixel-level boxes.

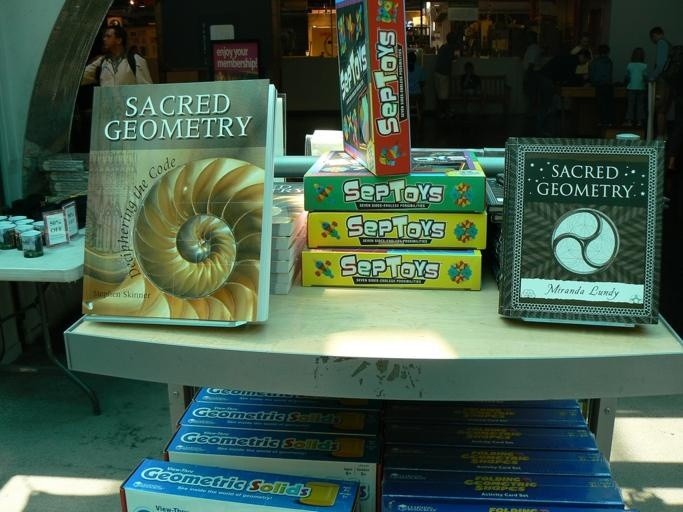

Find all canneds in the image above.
[0,215,44,258]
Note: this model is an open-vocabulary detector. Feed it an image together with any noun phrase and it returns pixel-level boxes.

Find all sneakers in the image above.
[622,119,642,128]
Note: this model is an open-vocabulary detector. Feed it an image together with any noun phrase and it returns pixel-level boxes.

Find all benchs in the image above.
[436,72,514,117]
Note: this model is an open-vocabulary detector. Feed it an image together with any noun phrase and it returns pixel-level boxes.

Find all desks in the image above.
[557,84,639,135]
[0,225,102,418]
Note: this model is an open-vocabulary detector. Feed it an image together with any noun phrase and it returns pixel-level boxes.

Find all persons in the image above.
[81,23,154,87]
[406,25,675,139]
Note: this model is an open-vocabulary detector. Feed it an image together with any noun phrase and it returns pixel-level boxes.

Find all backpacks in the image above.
[662,38,683,89]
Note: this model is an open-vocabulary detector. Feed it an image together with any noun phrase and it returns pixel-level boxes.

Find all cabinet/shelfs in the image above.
[474,10,543,57]
[59,283,682,474]
